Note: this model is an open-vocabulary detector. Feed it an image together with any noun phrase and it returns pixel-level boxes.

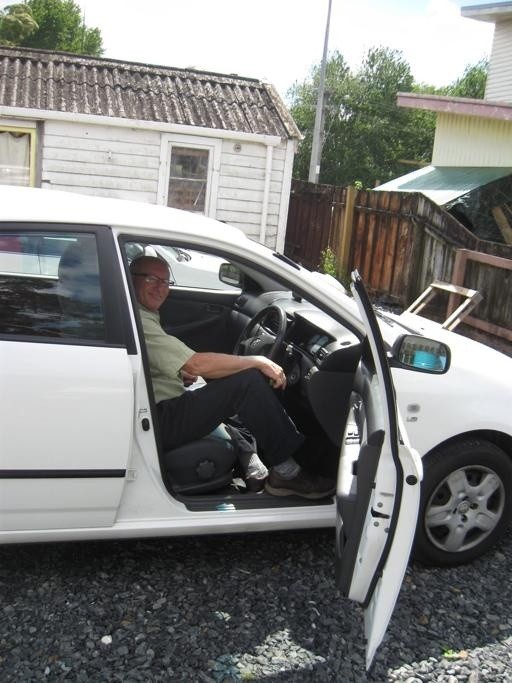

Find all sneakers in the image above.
[243,464,339,501]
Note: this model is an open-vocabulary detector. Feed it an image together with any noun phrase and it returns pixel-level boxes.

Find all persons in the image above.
[129,255,335,501]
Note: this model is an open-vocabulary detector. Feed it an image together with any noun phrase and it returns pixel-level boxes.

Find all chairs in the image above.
[156,419,249,497]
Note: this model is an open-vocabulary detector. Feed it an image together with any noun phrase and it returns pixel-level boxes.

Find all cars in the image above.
[1,186,512,673]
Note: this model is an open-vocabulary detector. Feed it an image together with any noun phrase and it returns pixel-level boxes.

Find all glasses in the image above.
[133,272,174,289]
